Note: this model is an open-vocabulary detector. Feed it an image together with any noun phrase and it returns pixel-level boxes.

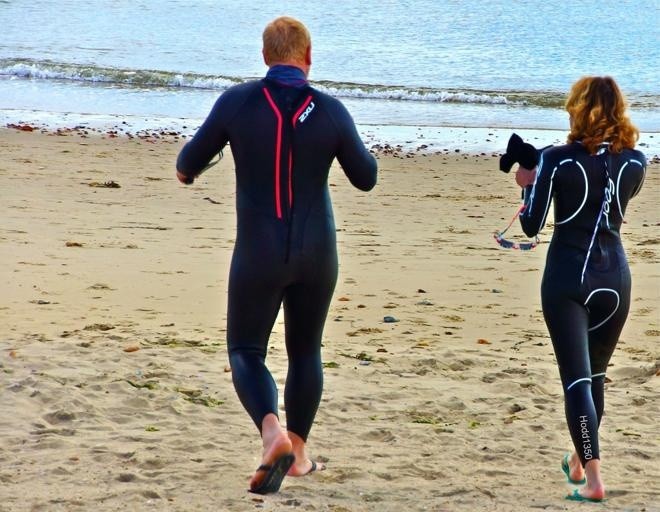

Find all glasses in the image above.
[493,204,541,251]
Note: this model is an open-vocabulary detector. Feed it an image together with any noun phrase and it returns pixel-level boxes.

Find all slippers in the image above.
[247,453,317,494]
[561,454,604,503]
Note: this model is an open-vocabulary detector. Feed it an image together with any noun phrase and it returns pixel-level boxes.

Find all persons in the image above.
[176,16,378,492]
[515,75,647,500]
